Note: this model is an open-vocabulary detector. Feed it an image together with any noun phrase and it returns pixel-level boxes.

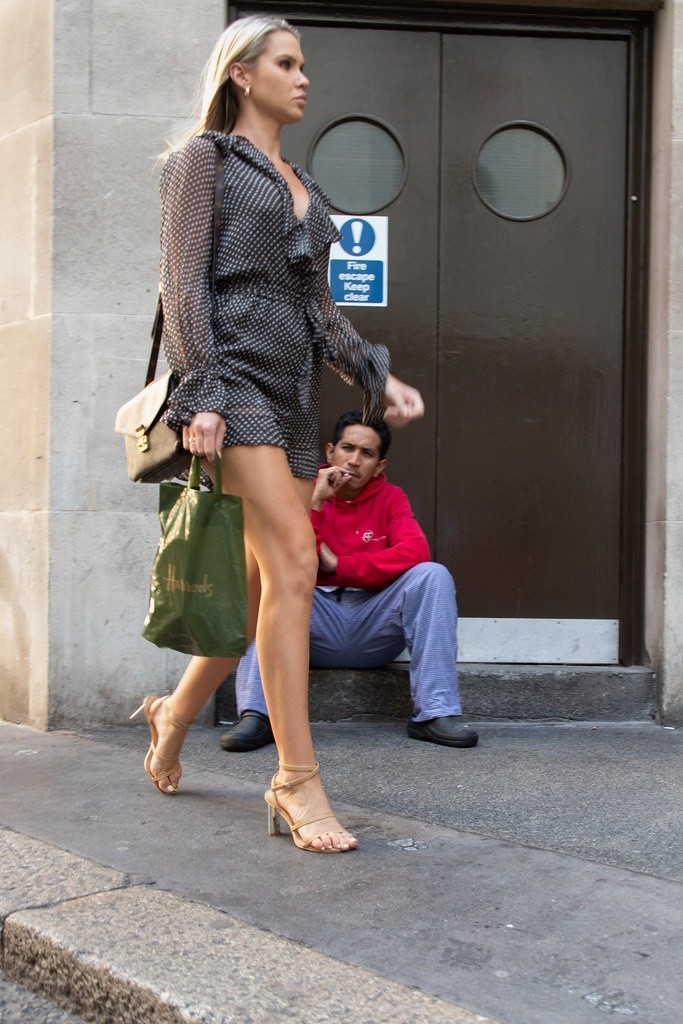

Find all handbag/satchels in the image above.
[142,450,247,657]
[113,370,197,485]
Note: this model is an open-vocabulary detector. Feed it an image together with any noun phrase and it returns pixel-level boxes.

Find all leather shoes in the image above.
[220,709,274,752]
[407,716,478,748]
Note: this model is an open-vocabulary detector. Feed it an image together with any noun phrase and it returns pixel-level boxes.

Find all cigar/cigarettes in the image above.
[342,473,349,477]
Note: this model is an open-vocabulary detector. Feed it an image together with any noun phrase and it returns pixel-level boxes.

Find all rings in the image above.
[189,437,196,442]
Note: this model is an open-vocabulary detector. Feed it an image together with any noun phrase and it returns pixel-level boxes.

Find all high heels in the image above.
[265,762,357,852]
[129,694,194,795]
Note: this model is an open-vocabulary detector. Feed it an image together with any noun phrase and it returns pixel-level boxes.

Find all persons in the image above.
[130,18,423,854]
[220,410,479,752]
